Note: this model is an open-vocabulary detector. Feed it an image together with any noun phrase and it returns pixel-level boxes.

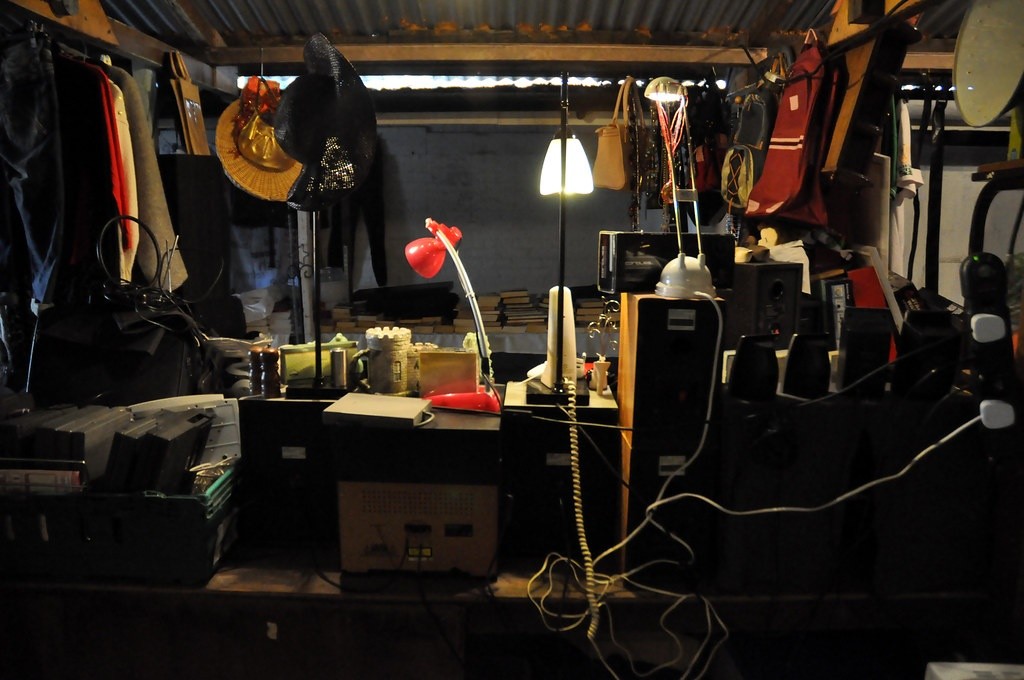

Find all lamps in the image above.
[644,76,718,300]
[404,217,502,418]
[525,71,595,405]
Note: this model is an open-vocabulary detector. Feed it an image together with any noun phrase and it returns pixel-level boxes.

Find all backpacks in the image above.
[745,28,841,224]
[721,52,776,208]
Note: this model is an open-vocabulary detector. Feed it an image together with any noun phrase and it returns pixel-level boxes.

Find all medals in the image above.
[661,182,680,204]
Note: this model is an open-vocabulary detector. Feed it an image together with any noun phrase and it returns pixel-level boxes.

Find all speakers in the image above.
[722,152,964,414]
[160,153,230,293]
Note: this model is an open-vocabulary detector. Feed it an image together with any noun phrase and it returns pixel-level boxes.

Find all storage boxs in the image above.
[0,465,243,591]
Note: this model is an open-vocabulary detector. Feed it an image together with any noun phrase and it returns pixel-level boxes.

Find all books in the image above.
[804,242,905,364]
[247,289,621,335]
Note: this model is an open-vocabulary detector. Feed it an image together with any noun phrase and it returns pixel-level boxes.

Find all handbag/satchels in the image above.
[166,49,210,156]
[591,77,652,190]
[36,214,223,409]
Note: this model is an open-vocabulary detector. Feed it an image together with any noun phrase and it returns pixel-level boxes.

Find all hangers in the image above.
[26,20,91,60]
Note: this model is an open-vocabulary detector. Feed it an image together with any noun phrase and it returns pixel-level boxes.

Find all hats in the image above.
[215,96,303,201]
